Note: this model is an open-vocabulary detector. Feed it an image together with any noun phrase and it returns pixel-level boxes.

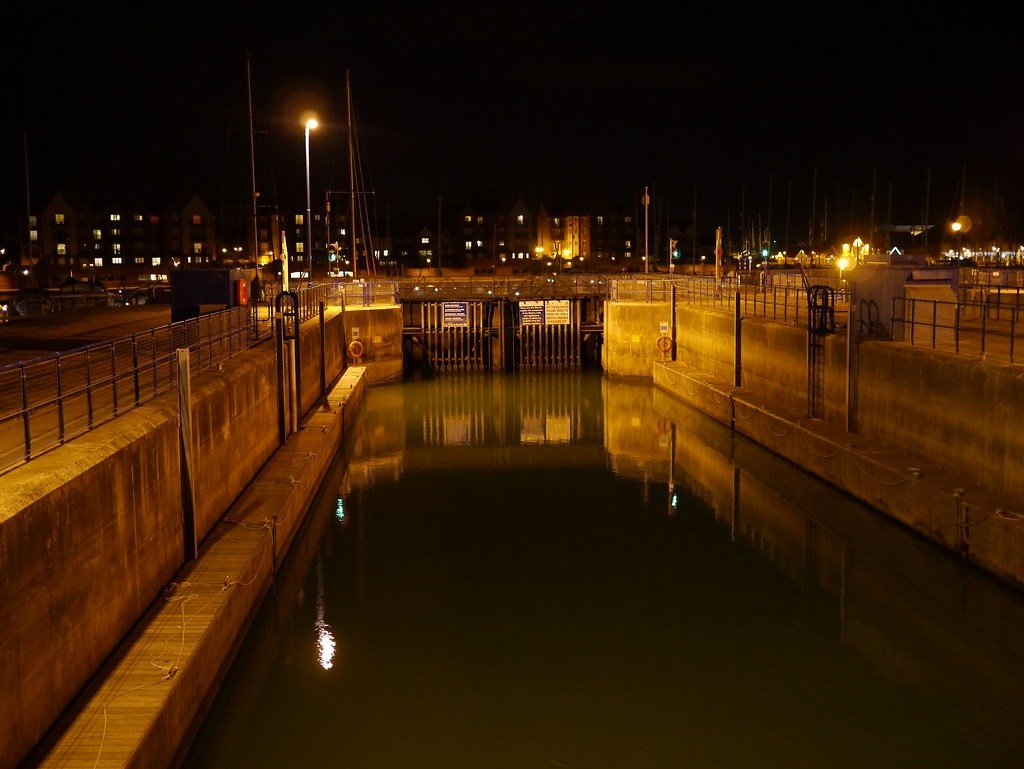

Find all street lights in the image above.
[303,118,318,309]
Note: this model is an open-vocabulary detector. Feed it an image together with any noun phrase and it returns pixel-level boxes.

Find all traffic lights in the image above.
[762,241,770,258]
[671,240,681,260]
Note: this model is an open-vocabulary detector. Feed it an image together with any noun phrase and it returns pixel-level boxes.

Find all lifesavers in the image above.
[656,335,672,351]
[349,341,364,358]
